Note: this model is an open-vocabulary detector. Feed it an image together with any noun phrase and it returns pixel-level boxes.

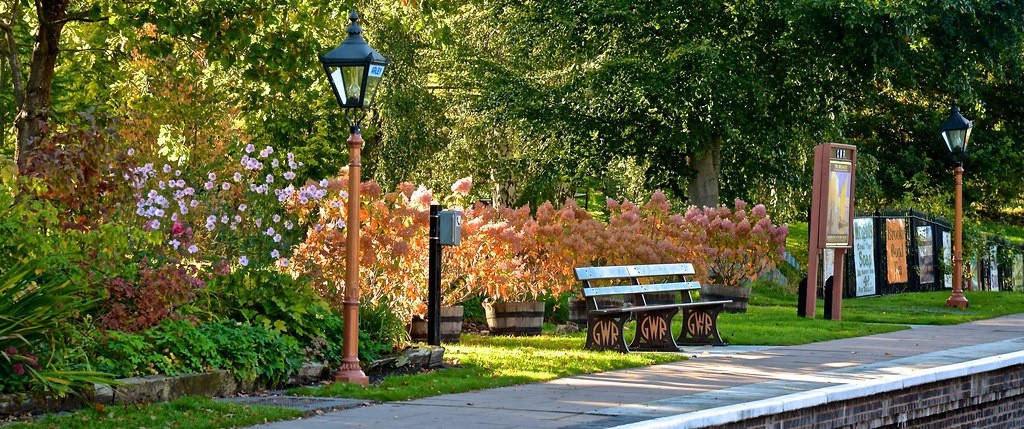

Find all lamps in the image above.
[936,100,974,167]
[322,8,388,134]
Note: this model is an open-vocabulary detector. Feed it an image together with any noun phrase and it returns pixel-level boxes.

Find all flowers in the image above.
[285,161,789,327]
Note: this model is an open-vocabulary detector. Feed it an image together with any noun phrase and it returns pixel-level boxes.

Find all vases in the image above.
[567,297,623,329]
[407,306,464,344]
[698,283,752,313]
[481,301,546,336]
[622,284,677,320]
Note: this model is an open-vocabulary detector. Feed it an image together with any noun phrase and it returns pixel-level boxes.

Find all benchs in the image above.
[572,262,734,356]
